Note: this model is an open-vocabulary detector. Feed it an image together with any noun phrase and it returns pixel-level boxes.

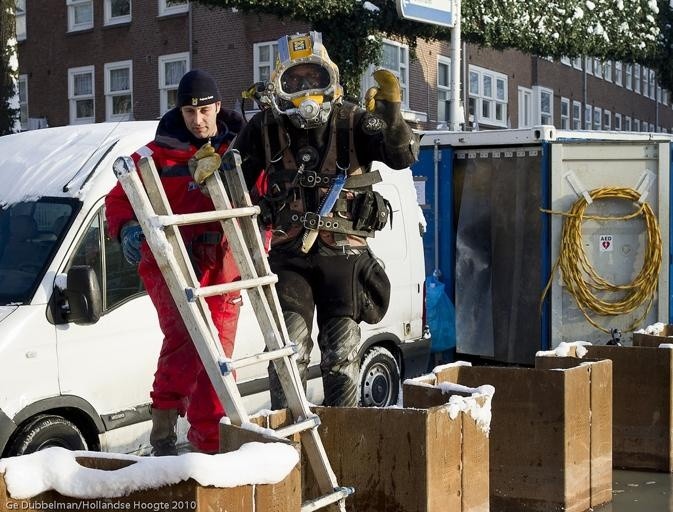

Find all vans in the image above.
[0,119,428,460]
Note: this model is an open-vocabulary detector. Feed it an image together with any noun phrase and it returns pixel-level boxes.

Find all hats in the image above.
[176,71,221,109]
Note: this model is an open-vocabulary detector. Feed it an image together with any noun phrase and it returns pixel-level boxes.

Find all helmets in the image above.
[261,30,339,130]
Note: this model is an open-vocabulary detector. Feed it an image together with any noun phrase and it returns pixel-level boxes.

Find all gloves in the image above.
[188,143,230,197]
[119,220,146,266]
[366,69,413,152]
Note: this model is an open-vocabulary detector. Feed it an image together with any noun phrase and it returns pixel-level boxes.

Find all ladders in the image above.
[112,148,356,512]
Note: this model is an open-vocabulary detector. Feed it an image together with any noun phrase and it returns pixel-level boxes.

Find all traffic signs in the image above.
[397,0,455,28]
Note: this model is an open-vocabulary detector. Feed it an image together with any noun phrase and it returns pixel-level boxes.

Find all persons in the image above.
[188,29,420,408]
[103,69,273,456]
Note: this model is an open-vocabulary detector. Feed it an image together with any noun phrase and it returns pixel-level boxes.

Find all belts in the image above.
[282,209,375,238]
[195,231,223,244]
[299,170,383,188]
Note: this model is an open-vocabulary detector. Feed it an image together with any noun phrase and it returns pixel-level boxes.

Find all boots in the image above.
[150,407,180,457]
